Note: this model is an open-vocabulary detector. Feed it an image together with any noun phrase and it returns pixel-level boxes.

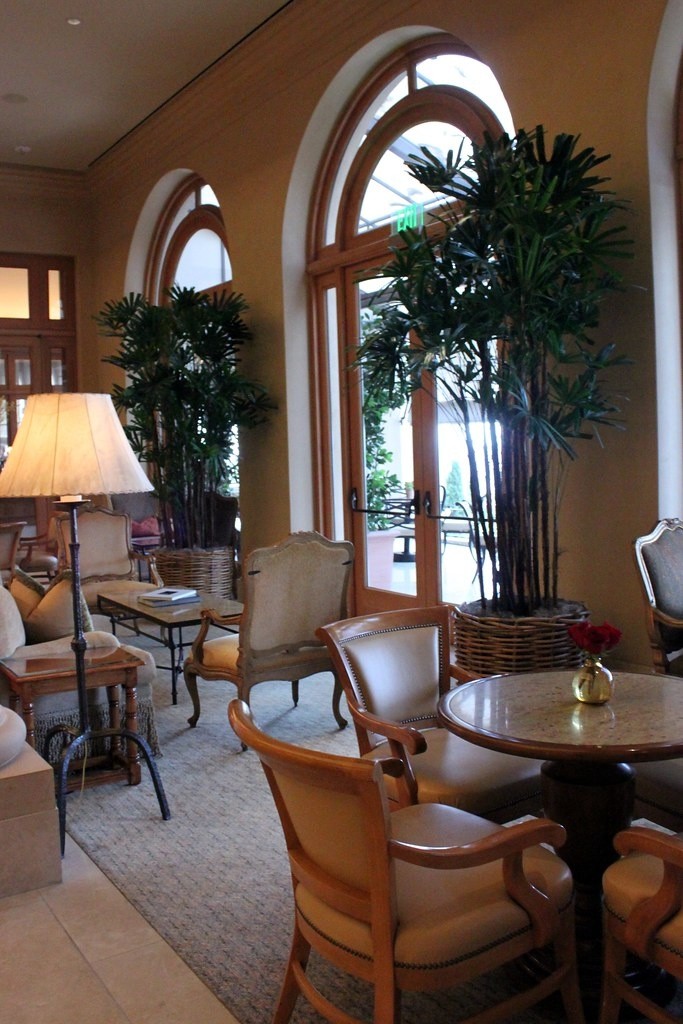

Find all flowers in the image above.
[567,620,625,664]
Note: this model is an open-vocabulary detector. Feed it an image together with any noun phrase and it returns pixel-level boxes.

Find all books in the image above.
[137,594,200,608]
[141,587,196,601]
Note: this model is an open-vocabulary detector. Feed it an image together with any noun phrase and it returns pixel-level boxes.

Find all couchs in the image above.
[0,586,170,775]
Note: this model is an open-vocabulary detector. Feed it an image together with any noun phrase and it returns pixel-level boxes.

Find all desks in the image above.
[382,498,415,562]
[96,587,244,705]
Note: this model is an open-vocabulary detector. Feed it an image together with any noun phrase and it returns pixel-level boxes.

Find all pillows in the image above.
[4,564,94,637]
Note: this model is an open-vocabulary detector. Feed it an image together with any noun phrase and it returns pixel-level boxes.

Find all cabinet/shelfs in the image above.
[435,668,683,1024]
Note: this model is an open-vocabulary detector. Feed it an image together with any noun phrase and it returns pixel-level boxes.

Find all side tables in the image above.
[0,646,147,796]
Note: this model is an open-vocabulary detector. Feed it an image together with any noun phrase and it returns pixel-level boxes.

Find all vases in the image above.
[571,656,616,704]
[571,703,615,741]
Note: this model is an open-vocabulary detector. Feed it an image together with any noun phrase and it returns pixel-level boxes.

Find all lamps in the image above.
[0,393,171,860]
[630,518,683,674]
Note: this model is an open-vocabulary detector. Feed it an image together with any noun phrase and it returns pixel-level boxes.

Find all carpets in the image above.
[66,630,683,1024]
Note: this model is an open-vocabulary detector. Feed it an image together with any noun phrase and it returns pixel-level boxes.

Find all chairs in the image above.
[316,607,545,824]
[229,704,584,1024]
[600,826,683,1024]
[455,500,497,583]
[207,491,242,579]
[55,504,164,615]
[15,515,60,584]
[183,530,356,729]
[442,494,486,563]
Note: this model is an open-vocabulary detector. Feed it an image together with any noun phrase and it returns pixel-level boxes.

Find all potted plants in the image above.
[337,126,653,687]
[364,450,402,590]
[404,482,414,499]
[92,284,279,600]
